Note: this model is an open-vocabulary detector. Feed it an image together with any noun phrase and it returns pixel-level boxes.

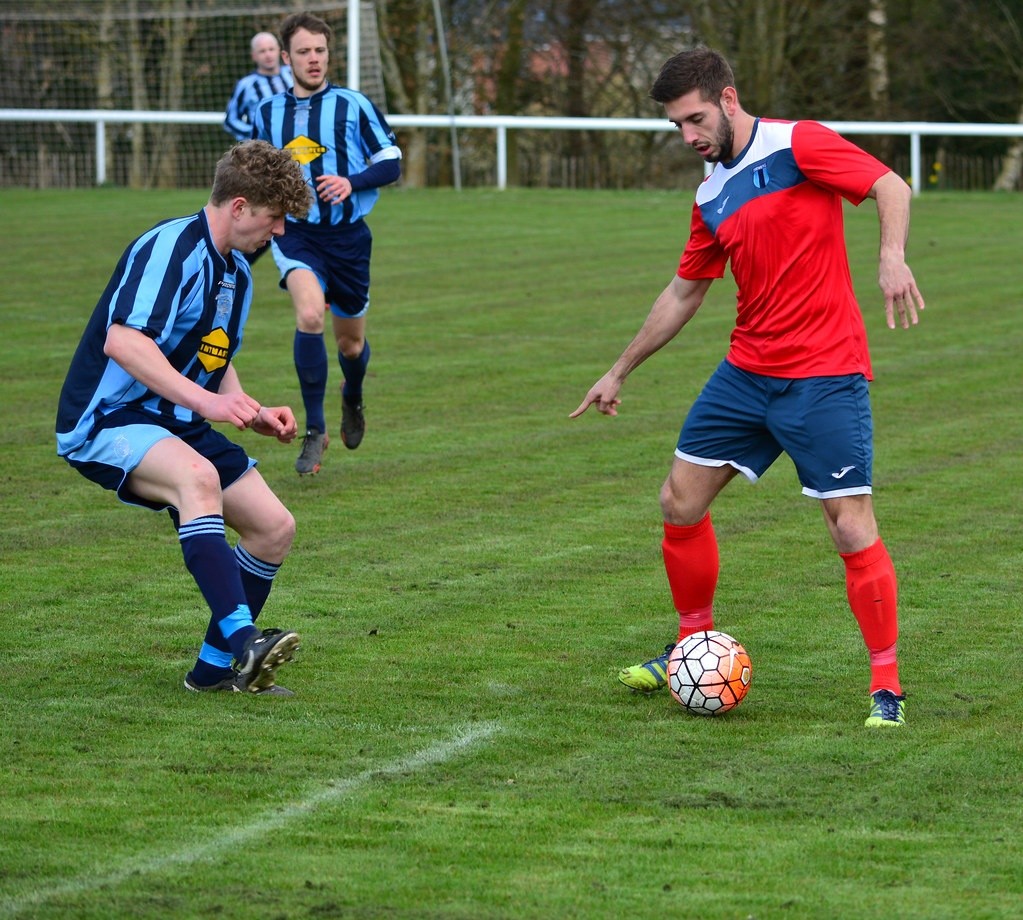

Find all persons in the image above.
[249,13,403,477]
[223,31,295,144]
[568,48,925,729]
[54,141,311,701]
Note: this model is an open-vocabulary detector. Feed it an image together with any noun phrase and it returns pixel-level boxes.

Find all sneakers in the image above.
[184,671,295,697]
[230,628,300,696]
[864,688,907,728]
[339,382,366,450]
[618,643,677,695]
[295,429,330,477]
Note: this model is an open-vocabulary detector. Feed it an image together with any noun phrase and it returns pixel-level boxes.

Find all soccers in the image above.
[666,629,753,716]
